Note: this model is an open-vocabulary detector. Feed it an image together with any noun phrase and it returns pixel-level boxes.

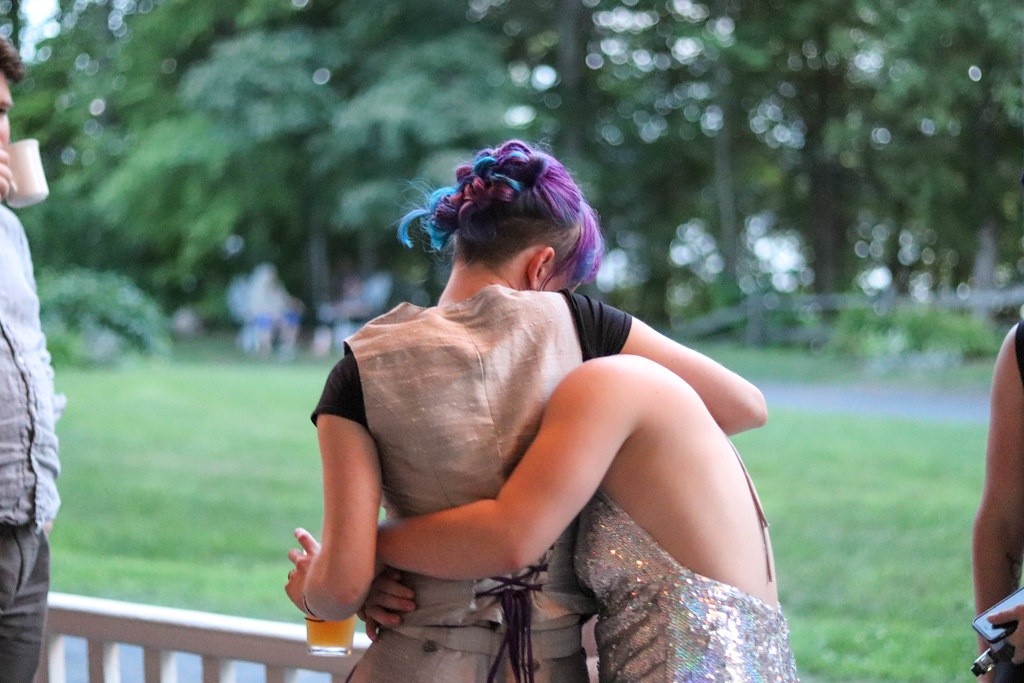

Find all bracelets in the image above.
[303,597,315,617]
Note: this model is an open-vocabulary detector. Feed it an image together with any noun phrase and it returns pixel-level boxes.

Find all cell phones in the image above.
[971,587,1024,643]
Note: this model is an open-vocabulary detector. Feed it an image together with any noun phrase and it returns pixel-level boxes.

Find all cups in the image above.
[4,138,49,208]
[303,543,356,656]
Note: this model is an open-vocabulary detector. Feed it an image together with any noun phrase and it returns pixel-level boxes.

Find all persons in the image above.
[973,321,1024,683]
[285,140,768,683]
[355,354,802,683]
[0,39,61,681]
[247,263,374,356]
